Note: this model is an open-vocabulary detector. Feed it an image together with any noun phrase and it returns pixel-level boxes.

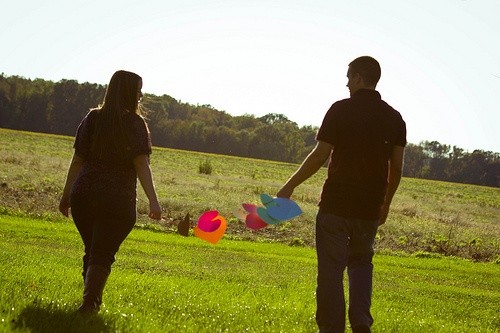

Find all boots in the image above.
[77,263,111,316]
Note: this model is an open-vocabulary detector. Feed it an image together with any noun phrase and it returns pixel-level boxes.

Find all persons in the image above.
[277,56,406,333]
[59,70,161,314]
